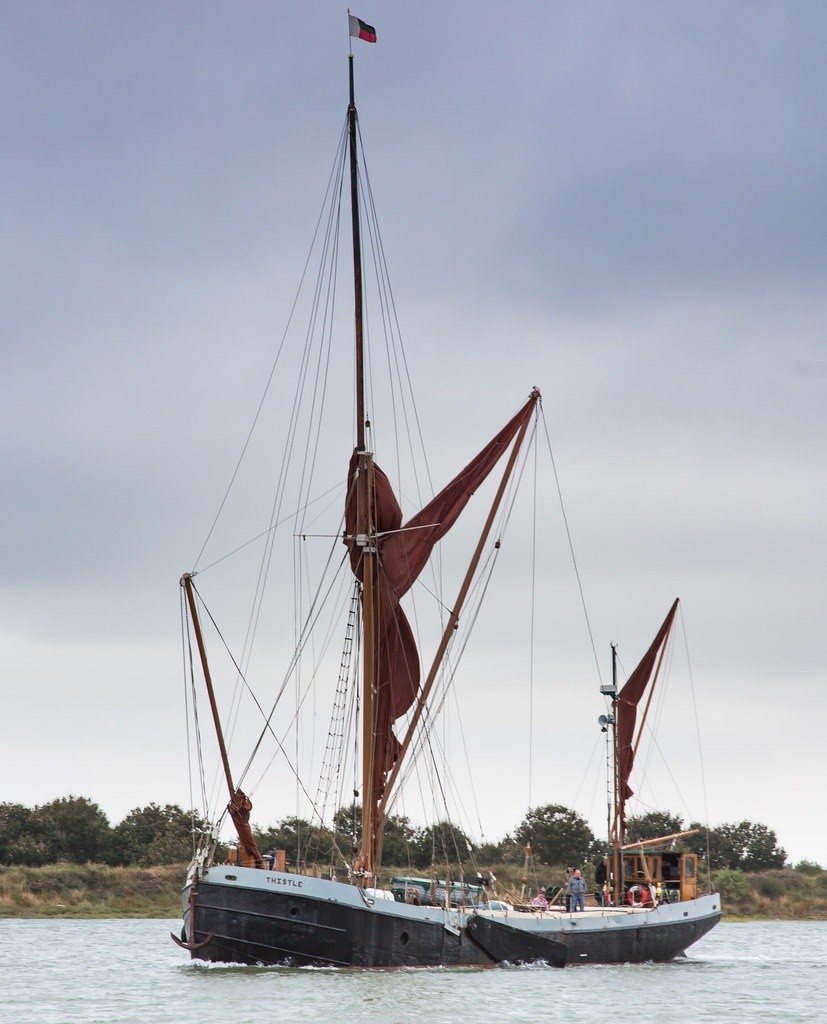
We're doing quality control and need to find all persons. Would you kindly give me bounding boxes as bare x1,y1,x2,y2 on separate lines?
532,889,548,911
594,860,607,896
565,867,586,913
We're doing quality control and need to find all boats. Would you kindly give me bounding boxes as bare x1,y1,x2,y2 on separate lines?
172,6,724,967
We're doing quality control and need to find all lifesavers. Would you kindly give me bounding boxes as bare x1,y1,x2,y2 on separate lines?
627,885,647,907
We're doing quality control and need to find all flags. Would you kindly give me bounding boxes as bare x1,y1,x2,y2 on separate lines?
349,14,378,44
